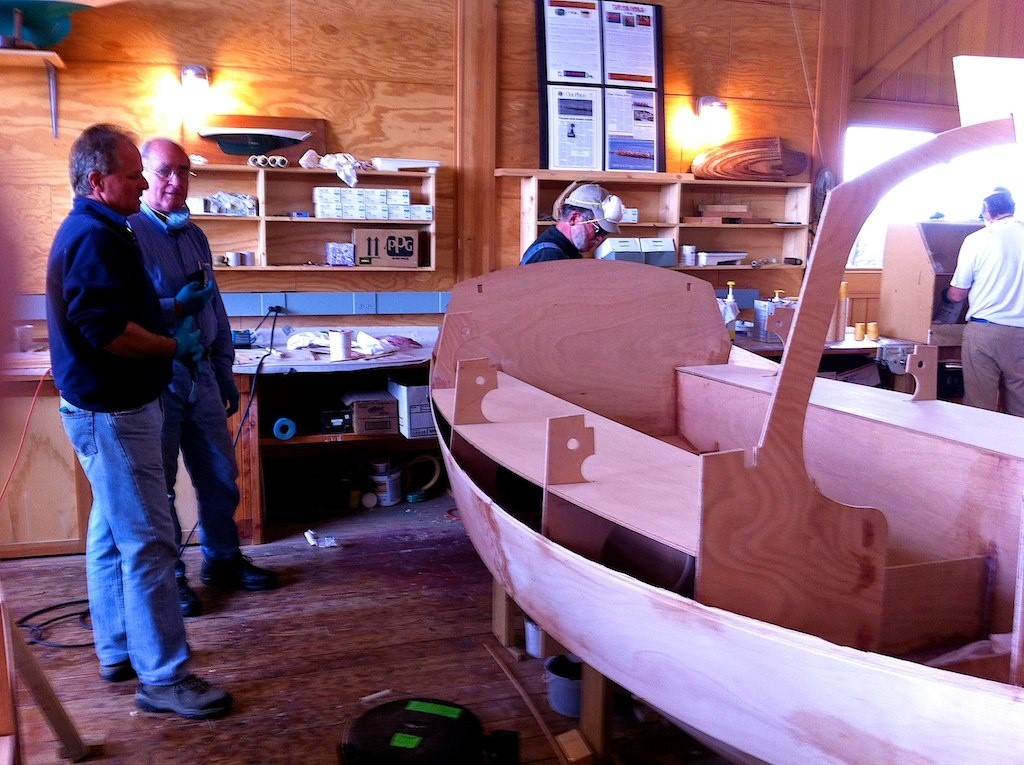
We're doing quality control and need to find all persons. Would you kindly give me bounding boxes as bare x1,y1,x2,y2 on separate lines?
47,122,235,720
944,183,1023,418
121,133,277,618
518,183,623,265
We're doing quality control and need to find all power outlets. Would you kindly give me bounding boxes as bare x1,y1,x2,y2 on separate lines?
353,292,376,314
260,292,286,316
439,292,453,313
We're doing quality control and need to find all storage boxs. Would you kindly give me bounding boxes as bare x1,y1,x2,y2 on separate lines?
351,390,399,435
387,377,438,440
352,226,418,268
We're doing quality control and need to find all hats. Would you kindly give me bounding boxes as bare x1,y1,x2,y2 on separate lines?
975,182,1009,216
565,183,622,234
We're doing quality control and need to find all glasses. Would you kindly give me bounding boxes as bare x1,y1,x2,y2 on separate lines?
577,211,608,235
143,168,197,181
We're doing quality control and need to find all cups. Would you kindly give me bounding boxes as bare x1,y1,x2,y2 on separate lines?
328,329,353,360
225,249,255,266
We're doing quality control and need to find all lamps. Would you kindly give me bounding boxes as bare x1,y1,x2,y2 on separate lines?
180,63,208,126
694,95,729,146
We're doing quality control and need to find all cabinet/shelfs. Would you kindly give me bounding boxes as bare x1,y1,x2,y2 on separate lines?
521,174,811,271
188,162,435,272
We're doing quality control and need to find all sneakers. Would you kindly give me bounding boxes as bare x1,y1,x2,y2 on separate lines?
175,576,203,617
98,642,192,683
199,557,280,591
134,675,235,719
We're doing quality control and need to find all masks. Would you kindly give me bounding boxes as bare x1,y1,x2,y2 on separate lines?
561,194,624,223
983,218,991,226
150,203,188,229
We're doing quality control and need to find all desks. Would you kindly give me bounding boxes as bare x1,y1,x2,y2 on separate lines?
2,342,434,558
724,324,926,396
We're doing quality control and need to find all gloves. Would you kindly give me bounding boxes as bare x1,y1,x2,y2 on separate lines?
172,314,202,358
941,288,952,303
189,343,203,372
219,380,239,417
174,279,215,317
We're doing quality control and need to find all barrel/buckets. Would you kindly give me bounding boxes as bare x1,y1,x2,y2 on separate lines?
368,467,403,507
